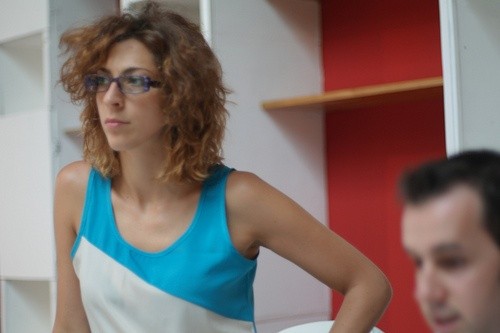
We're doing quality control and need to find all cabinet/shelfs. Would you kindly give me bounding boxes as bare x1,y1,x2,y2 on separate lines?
0,0,499,332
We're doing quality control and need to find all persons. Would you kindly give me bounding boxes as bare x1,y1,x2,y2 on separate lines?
50,4,393,331
395,149,500,333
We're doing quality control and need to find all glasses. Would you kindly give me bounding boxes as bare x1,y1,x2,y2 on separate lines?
83,74,163,94
